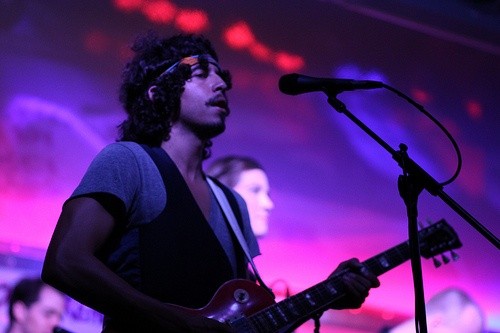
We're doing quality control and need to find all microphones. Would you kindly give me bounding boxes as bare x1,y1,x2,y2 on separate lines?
278,72,383,95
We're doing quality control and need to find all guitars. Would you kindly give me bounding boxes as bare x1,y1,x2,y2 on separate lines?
102,217,464,333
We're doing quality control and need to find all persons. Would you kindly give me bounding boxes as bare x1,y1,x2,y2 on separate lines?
41,34,380,333
208,155,274,236
8,280,64,333
381,288,482,333
